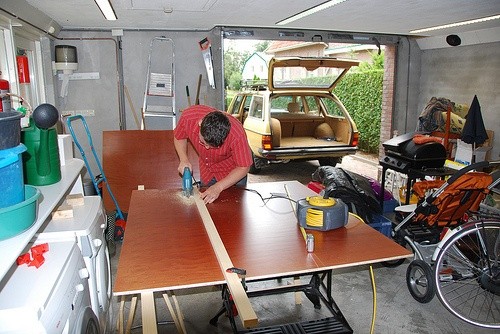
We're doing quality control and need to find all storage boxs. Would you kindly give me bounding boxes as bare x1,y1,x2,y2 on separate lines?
361,197,399,239
454,129,494,167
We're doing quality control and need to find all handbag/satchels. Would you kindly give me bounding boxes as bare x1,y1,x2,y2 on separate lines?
363,174,394,200
314,165,381,215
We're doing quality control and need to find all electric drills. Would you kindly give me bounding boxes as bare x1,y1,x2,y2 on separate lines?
184,167,194,198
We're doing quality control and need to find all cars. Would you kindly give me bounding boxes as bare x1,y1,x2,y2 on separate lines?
227,56,361,175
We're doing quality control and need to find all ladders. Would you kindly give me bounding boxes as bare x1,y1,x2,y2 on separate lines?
140,36,176,129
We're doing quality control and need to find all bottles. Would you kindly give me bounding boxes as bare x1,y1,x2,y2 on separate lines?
307,233,314,253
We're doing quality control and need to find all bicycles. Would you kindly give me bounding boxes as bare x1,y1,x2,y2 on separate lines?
432,218,499,330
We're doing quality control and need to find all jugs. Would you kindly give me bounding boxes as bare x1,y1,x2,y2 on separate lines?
20,103,62,186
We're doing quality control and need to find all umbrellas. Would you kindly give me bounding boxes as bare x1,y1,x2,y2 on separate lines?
461,95,488,143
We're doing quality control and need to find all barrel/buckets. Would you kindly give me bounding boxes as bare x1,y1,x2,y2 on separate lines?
0,143,28,209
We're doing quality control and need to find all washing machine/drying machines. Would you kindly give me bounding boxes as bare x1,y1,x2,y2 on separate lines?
0,193,114,334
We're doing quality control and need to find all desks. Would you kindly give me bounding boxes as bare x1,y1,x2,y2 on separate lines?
0,135,85,294
112,180,414,334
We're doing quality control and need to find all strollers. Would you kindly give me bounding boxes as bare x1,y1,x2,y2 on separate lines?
382,160,499,304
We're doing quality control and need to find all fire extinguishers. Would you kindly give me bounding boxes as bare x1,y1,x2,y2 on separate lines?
0,72,13,113
17,46,32,83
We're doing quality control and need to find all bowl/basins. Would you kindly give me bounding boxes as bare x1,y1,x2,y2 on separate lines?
0,184,41,242
0,110,22,150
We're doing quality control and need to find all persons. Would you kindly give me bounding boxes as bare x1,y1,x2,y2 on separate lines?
174,105,253,204
